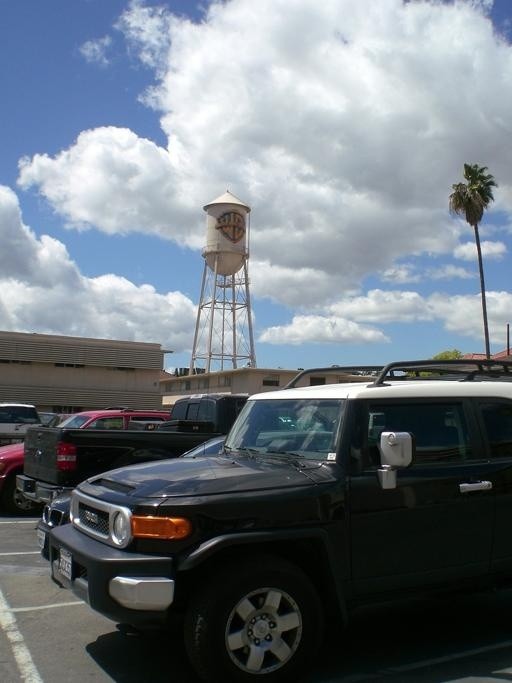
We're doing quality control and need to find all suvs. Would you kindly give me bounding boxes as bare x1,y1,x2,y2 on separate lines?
1,403,41,447
45,358,512,682
0,408,177,518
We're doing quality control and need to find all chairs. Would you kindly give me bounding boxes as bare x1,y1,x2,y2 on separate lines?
349,410,460,455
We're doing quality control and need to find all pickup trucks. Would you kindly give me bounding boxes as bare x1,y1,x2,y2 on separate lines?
14,393,261,508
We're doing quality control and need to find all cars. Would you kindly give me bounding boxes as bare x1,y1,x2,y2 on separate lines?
37,412,76,428
